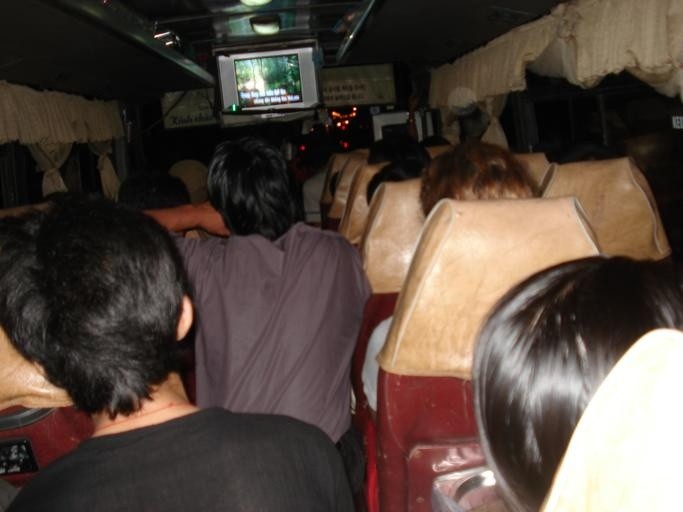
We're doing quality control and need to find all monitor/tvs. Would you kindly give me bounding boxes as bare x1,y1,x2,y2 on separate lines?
216,46,320,113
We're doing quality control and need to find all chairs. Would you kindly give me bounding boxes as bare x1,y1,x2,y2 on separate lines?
0,142,682,511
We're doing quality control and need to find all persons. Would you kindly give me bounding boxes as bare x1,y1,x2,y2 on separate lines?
1,136,373,511
470,255,683,512
361,135,537,413
